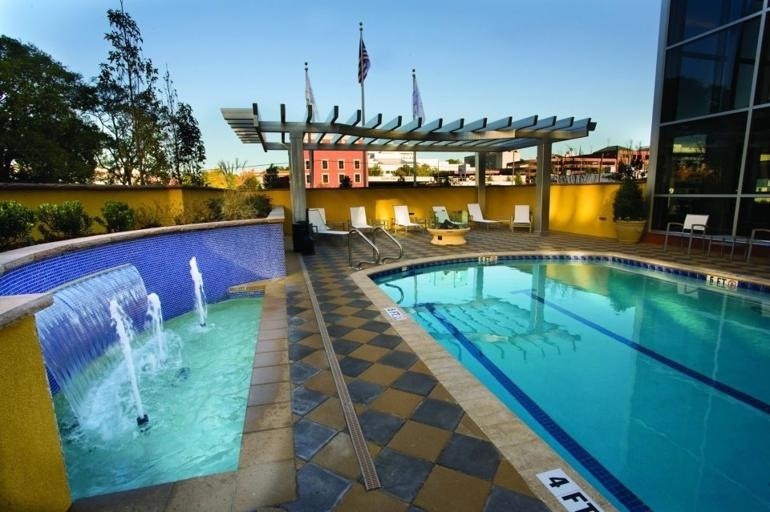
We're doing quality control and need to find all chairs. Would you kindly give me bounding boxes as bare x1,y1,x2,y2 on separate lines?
664,214,710,259
307,203,537,246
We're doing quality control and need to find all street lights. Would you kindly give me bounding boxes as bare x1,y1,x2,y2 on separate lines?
512,150,517,183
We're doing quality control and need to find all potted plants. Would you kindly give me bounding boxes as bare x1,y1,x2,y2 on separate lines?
609,175,648,244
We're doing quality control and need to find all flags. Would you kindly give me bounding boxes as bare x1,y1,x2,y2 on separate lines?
357,32,370,84
412,78,426,126
304,73,319,123
